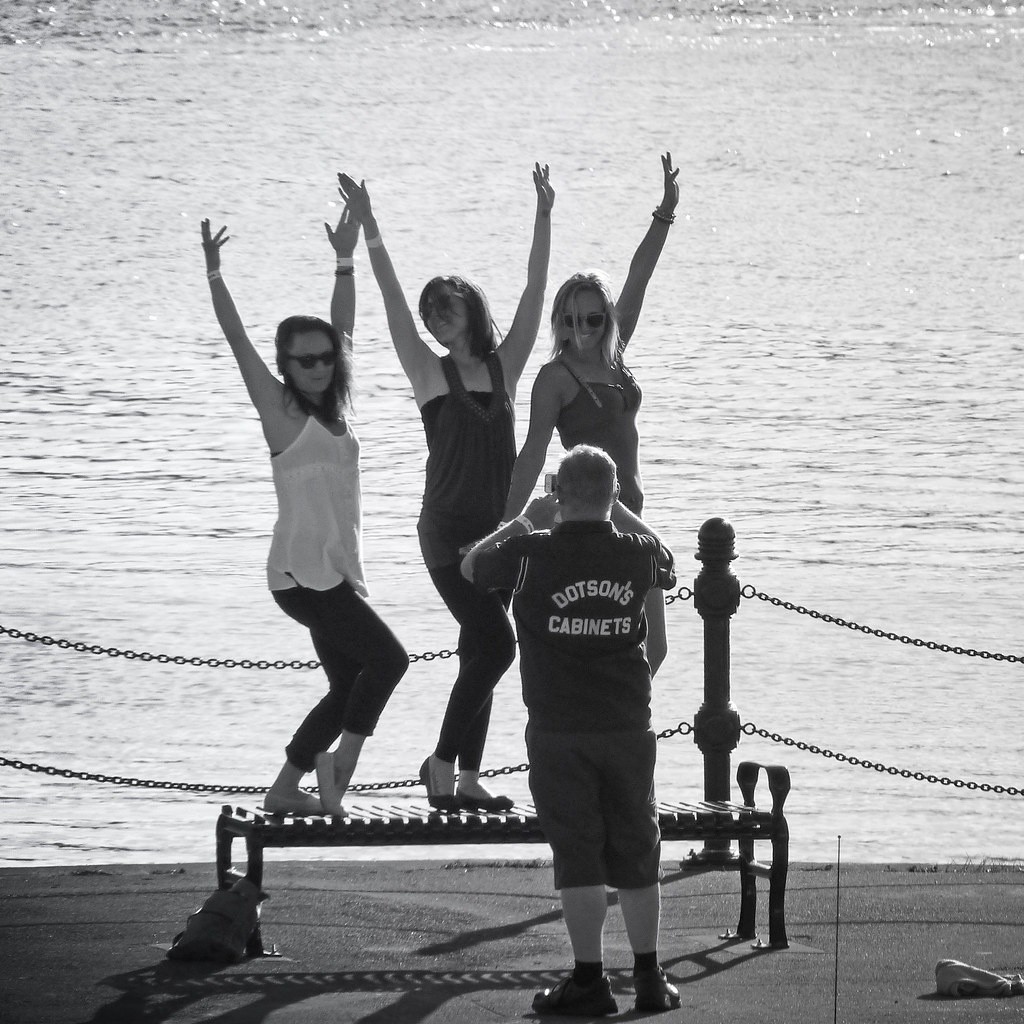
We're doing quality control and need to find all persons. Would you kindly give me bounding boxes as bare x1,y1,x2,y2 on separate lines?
459,445,684,1016
201,204,408,815
453,152,678,680
338,159,556,813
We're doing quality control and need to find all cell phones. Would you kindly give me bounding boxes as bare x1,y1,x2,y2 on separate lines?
544,474,556,493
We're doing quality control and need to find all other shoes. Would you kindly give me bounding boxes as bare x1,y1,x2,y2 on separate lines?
454,788,514,811
419,756,455,809
264,791,327,813
317,751,348,816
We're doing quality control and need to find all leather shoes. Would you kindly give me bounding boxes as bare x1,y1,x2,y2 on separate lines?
533,976,619,1015
635,967,683,1012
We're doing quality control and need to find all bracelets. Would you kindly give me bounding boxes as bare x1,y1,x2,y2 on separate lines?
651,206,676,224
207,270,222,281
333,257,355,277
515,515,534,535
366,234,384,248
497,521,507,529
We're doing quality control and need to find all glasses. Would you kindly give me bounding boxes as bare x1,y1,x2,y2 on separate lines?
560,312,607,329
419,291,467,320
287,351,339,370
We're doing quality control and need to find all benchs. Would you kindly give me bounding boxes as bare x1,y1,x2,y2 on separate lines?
215,762,790,958
